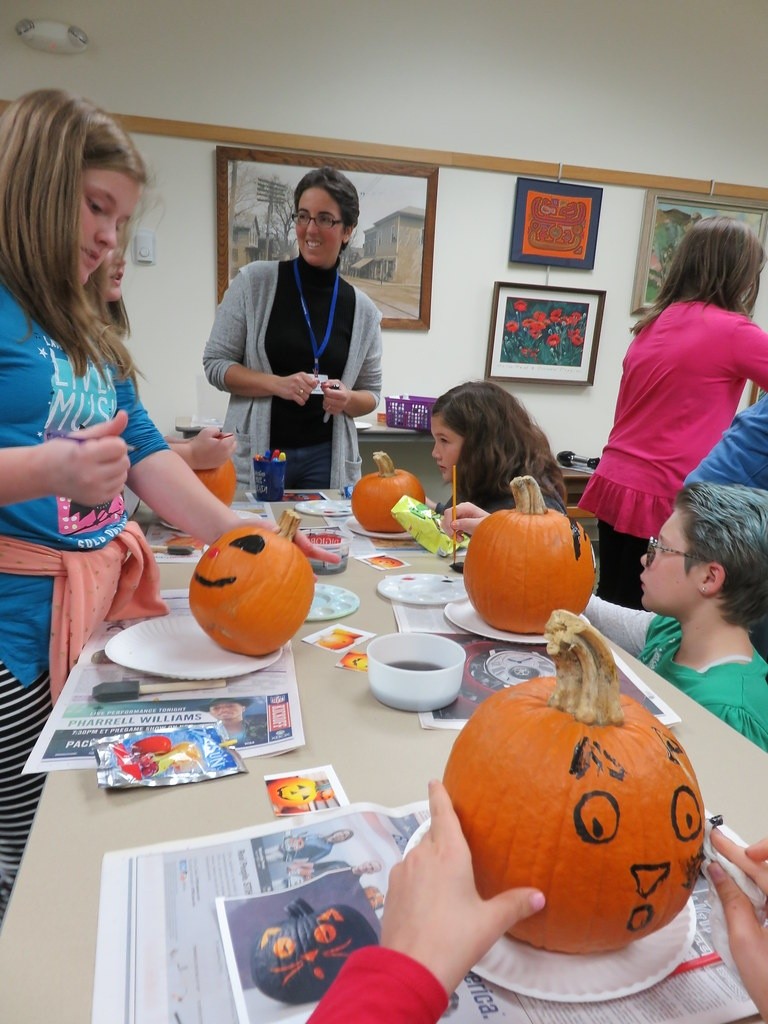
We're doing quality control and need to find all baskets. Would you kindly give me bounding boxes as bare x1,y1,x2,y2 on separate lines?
384,395,438,433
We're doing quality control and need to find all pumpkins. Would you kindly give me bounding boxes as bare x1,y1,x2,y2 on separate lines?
188,508,315,656
440,608,707,956
463,476,597,636
351,451,426,533
193,458,235,507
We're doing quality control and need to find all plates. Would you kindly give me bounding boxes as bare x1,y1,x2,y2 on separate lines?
305,583,360,623
226,509,264,525
293,497,352,518
444,599,556,646
377,571,473,606
346,510,416,541
469,894,695,1004
99,611,288,684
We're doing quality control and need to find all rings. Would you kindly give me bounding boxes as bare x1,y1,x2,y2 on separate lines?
299,390,303,394
326,405,331,410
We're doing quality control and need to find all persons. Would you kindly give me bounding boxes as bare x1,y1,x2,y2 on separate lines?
0,88,342,922
304,781,768,1024
203,168,384,490
265,827,382,891
578,221,768,612
199,698,269,751
100,244,134,340
683,393,768,491
423,380,567,545
440,483,768,752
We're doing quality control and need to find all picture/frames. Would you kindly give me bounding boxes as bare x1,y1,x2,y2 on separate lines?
633,194,768,321
509,177,603,268
206,147,440,329
485,282,608,387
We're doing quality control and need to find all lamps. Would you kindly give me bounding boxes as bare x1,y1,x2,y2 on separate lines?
14,19,88,55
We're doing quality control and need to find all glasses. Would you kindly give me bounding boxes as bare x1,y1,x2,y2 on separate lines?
646,536,710,567
292,213,343,229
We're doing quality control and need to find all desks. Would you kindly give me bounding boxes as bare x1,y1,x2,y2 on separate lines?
163,419,435,444
0,486,768,1024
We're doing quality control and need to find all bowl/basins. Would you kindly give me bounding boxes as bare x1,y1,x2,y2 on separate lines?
355,629,469,714
